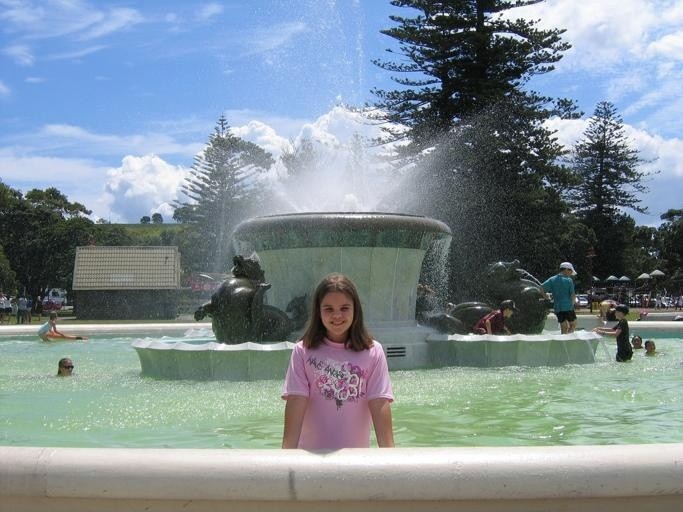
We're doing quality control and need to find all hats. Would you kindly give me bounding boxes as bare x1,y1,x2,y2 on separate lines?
610,304,629,314
500,300,520,312
560,262,578,275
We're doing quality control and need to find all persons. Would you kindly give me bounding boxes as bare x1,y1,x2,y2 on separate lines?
588,304,634,363
472,299,521,335
588,284,683,313
538,261,579,334
631,334,644,350
36,311,65,344
279,270,395,449
57,358,76,377
645,340,658,357
0,292,44,324
